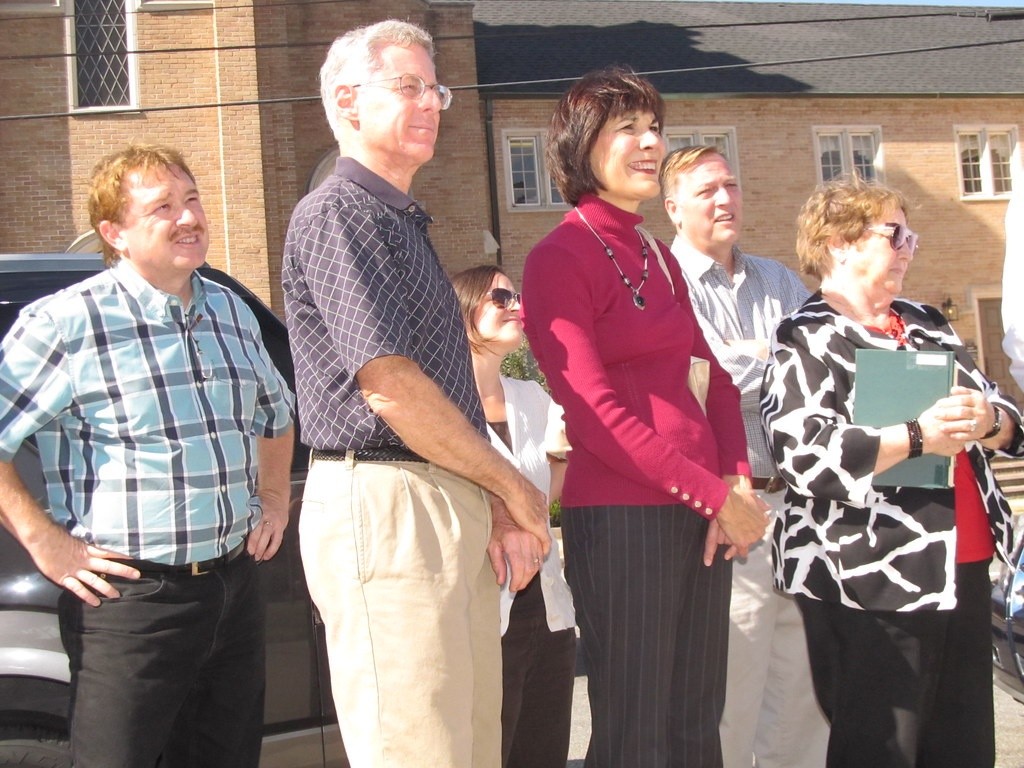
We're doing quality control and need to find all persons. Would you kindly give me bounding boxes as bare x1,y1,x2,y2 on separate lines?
279,18,553,768
756,170,1024,768
659,142,830,768
0,142,297,768
521,64,773,768
443,266,571,768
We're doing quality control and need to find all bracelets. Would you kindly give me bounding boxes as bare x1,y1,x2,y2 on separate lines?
903,415,925,460
978,400,1004,439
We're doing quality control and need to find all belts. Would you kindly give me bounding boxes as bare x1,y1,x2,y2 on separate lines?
106,539,246,576
311,445,430,463
752,476,788,494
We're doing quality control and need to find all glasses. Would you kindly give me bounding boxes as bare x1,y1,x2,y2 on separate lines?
351,74,453,110
862,223,919,251
484,288,520,309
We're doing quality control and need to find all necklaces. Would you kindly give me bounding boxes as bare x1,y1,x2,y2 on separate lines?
573,205,651,314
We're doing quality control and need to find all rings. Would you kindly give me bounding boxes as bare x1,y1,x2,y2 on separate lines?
533,557,541,564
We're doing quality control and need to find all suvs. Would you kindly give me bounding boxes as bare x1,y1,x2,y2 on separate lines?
0,251,345,768
